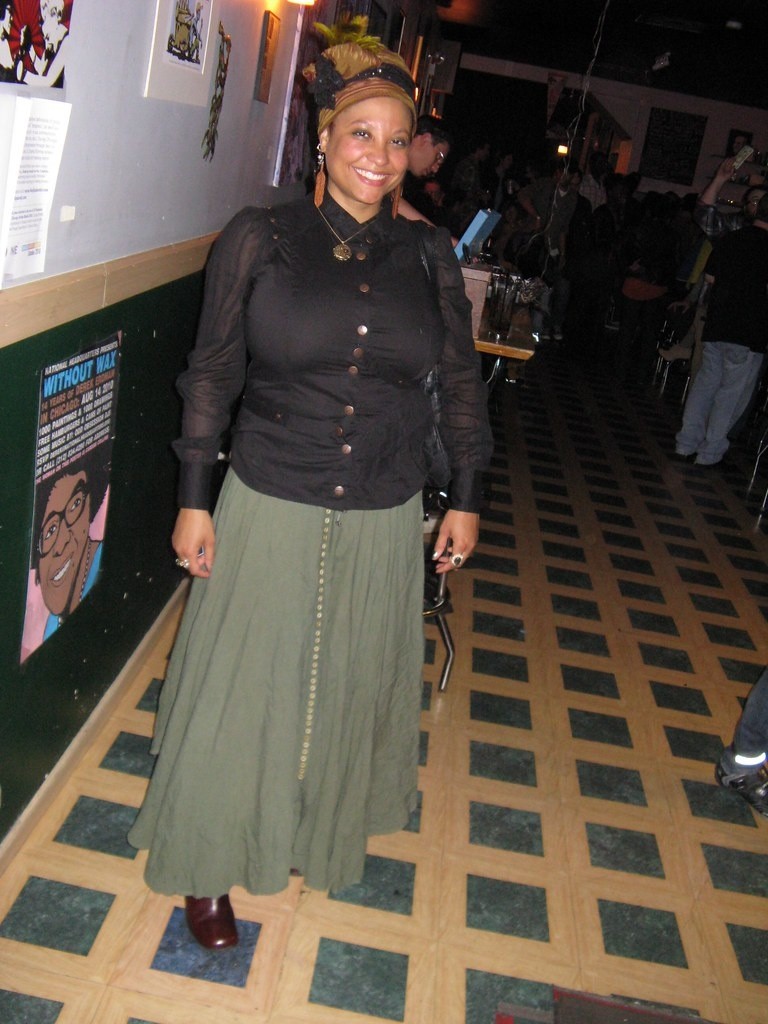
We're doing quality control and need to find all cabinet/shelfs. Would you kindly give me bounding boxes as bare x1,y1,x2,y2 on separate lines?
705,152,767,207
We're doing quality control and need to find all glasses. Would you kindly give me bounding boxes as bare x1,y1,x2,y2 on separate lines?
743,198,759,206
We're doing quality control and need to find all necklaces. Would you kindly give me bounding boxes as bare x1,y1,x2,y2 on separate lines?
316,202,380,261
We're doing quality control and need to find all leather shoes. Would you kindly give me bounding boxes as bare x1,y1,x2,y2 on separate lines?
184,896,237,950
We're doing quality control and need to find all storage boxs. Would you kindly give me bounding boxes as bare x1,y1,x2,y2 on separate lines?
454,207,502,339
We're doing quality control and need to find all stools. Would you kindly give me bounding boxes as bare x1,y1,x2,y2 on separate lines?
424,513,455,692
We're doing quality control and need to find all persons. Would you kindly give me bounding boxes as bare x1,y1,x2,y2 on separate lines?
128,35,496,953
666,190,767,472
404,139,725,382
400,112,463,263
689,158,768,388
714,668,767,817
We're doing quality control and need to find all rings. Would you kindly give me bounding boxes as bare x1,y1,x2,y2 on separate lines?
451,553,463,567
176,559,189,568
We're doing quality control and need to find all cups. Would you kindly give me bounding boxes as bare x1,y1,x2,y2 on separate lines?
507,179,517,195
489,272,521,336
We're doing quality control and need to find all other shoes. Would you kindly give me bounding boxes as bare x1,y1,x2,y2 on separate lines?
716,758,768,817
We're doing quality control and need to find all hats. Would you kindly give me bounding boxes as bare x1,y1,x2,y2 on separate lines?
302,42,417,138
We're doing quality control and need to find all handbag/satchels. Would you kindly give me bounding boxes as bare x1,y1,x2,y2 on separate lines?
424,367,453,486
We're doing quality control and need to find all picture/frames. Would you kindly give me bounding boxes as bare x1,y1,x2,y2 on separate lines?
367,0,387,46
725,128,754,157
252,10,281,105
390,7,407,56
143,0,222,106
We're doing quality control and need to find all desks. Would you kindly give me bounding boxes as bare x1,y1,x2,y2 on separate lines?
474,305,534,417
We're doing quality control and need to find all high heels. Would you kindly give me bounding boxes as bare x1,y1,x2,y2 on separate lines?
657,343,691,368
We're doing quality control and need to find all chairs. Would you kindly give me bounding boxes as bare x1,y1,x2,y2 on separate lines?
652,305,768,511
